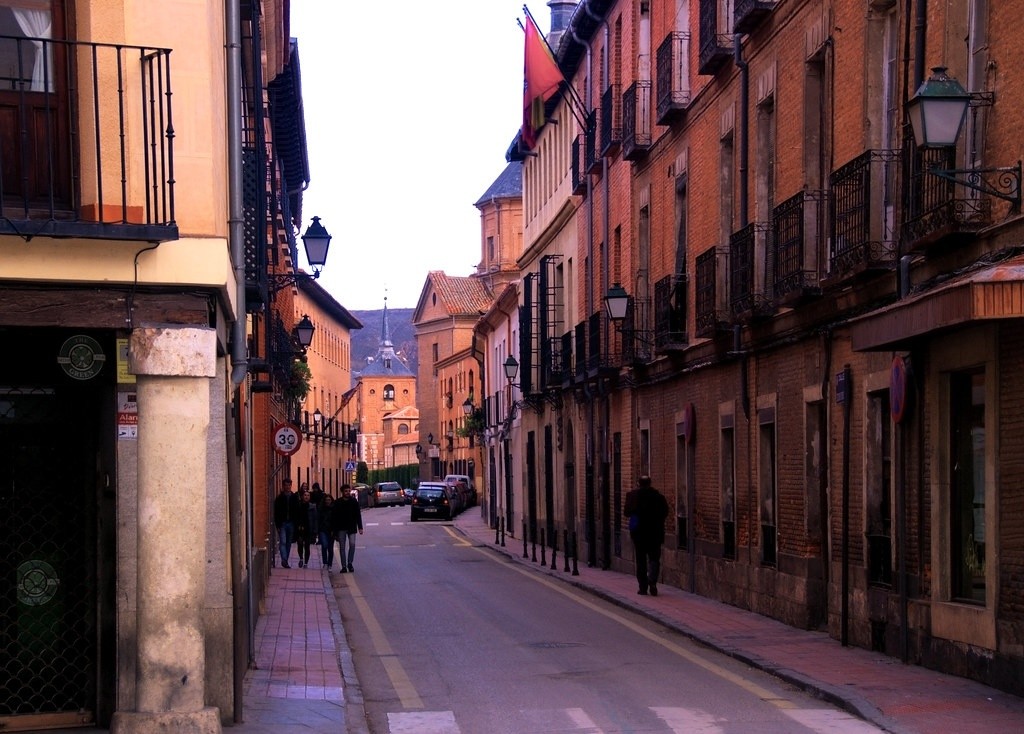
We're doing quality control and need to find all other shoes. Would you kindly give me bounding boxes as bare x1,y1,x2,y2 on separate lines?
281,560,291,568
648,580,657,596
347,563,354,572
637,590,647,595
328,566,332,573
316,541,320,545
304,565,308,569
340,566,348,573
323,564,329,573
299,559,303,568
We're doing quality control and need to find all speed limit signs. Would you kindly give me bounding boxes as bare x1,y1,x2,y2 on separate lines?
271,421,303,458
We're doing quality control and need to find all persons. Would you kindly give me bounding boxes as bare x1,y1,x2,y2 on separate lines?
309,481,326,546
330,484,364,574
316,493,337,571
272,477,299,568
622,475,669,597
292,490,318,569
294,482,311,509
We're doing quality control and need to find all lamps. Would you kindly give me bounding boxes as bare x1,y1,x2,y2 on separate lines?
416,443,426,454
278,314,316,351
462,398,481,419
428,433,440,445
348,419,360,434
905,67,1023,208
268,215,333,294
503,354,536,392
448,426,457,437
302,408,321,427
604,281,667,346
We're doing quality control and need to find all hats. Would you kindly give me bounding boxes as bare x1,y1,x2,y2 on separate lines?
312,483,319,489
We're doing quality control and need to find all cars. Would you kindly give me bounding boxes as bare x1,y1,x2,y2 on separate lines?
368,481,405,508
403,473,477,523
